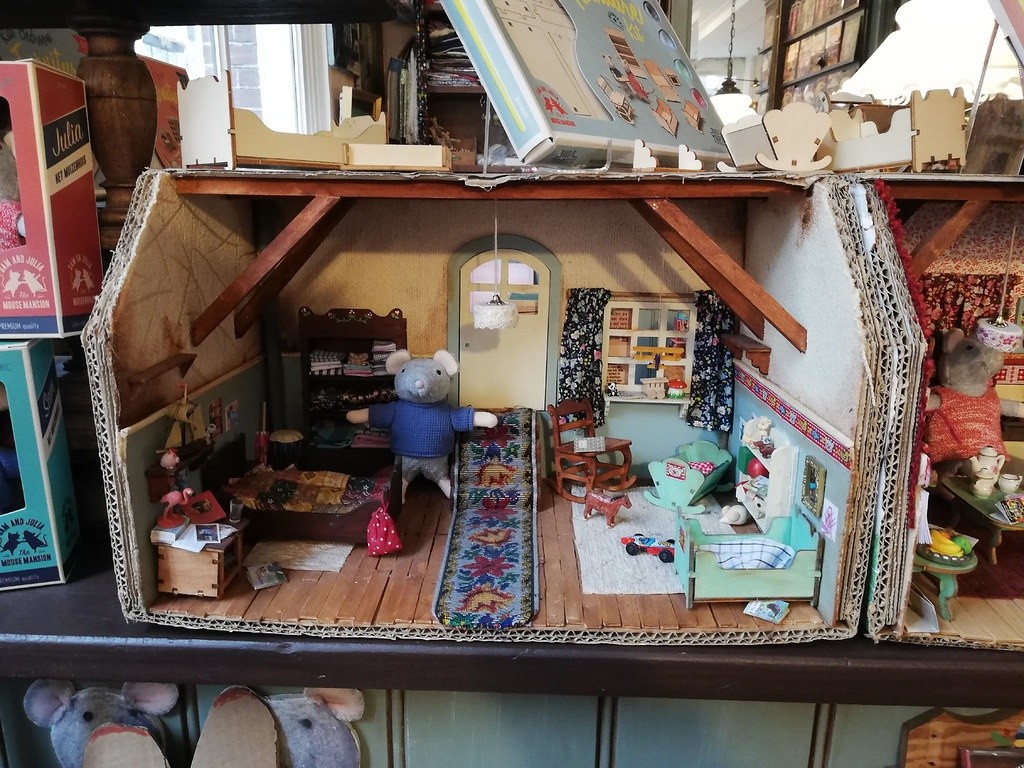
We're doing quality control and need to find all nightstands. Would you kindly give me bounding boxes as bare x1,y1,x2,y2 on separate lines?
152,518,251,599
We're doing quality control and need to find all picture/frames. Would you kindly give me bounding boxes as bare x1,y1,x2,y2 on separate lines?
801,455,827,518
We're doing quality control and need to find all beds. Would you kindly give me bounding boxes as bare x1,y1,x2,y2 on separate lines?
674,505,826,610
644,440,734,515
200,433,402,544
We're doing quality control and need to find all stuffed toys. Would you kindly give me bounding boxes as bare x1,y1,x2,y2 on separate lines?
161,451,181,469
346,349,499,504
927,329,1024,481
23,679,364,768
736,480,767,519
606,382,618,397
742,417,770,448
719,506,749,525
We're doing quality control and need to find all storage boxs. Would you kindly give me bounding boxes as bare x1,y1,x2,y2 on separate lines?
0,342,84,591
439,0,738,174
1,58,106,339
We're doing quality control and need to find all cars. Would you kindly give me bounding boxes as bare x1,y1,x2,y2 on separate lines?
620,532,675,564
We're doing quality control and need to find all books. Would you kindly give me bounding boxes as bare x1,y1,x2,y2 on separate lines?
151,515,190,540
246,561,288,590
743,600,790,625
574,437,605,453
990,498,1024,525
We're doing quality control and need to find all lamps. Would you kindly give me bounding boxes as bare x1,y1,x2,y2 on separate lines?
475,199,521,330
973,201,1023,354
706,1,752,122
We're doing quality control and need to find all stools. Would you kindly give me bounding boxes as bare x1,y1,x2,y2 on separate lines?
912,553,978,621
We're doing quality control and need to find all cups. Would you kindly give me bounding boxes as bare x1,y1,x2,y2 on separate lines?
999,474,1023,493
971,469,994,498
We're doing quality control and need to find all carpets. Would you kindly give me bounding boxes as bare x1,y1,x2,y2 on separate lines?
242,539,355,573
432,407,540,630
570,484,738,595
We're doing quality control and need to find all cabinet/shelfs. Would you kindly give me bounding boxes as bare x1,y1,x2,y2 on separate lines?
736,416,799,535
417,3,526,174
297,306,407,468
756,0,902,116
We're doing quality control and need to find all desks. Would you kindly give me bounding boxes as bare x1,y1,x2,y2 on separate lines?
937,476,1024,564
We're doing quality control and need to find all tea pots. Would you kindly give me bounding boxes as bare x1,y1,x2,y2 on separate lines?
968,446,1005,485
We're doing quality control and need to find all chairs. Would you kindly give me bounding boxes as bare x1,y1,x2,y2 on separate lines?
546,398,637,502
